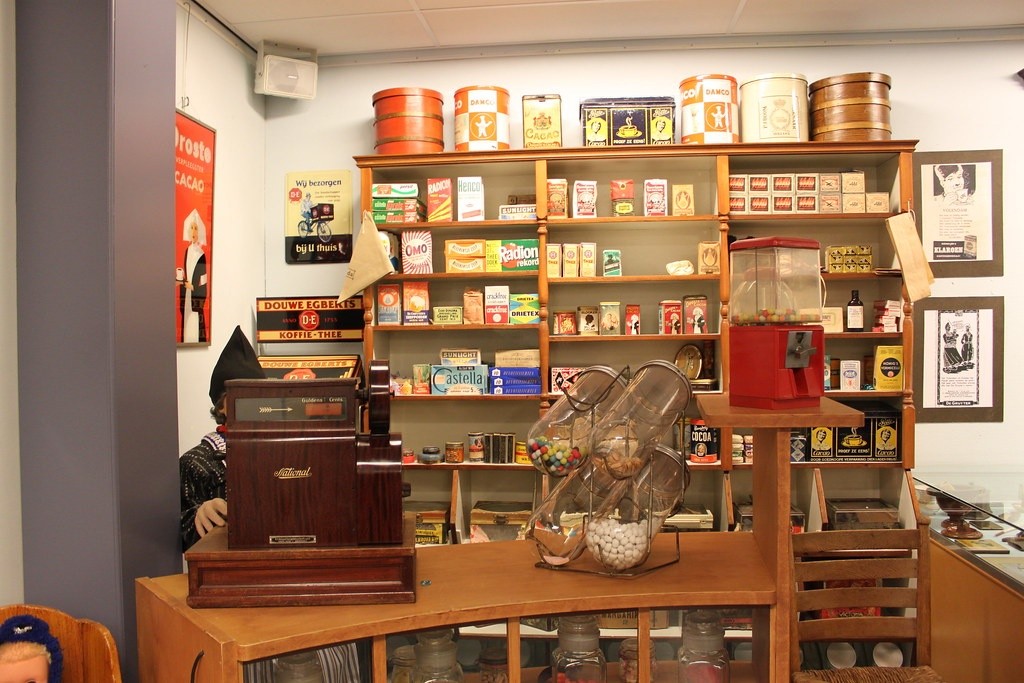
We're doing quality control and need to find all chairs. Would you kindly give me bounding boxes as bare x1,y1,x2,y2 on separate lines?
785,516,945,682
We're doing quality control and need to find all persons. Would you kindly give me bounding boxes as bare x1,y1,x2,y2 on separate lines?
179,325,360,683
181,208,207,341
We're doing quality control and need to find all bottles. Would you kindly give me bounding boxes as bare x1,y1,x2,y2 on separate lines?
273,651,325,683
548,613,732,683
389,631,508,683
846,289,865,332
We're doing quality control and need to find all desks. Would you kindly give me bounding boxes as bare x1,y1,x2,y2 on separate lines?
138,528,775,683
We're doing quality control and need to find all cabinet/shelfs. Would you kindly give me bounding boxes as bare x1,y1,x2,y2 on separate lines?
350,142,927,640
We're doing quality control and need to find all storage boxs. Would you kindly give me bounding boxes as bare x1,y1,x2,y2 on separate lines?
579,96,676,146
374,171,917,629
523,95,563,147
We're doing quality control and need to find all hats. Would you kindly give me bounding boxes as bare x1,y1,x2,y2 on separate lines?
209,326,267,407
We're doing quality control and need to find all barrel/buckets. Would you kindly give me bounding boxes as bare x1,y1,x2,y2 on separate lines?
371,87,445,154
452,85,510,152
806,72,894,142
677,73,742,145
738,72,812,143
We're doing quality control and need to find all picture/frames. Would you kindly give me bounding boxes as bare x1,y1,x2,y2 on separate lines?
912,292,1005,423
172,108,216,346
911,146,1005,279
